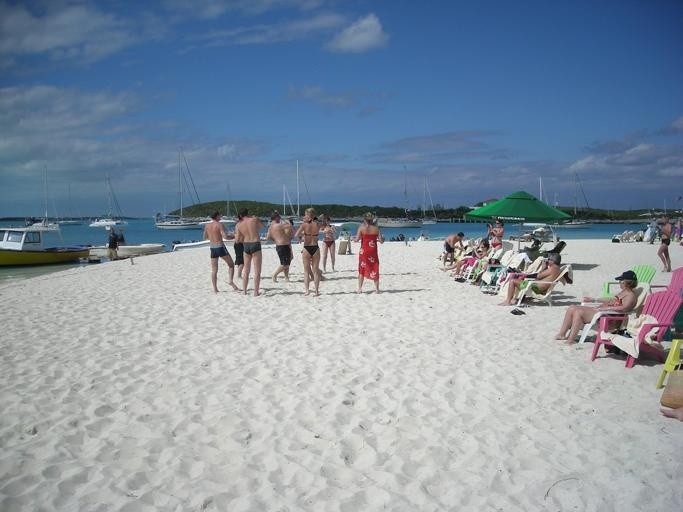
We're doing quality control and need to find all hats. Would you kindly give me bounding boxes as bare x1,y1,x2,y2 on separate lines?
615,271,637,280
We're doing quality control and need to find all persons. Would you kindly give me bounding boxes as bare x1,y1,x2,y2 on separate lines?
204,210,238,293
649,213,683,241
264,220,294,241
352,212,385,295
322,218,337,274
439,220,561,306
655,215,672,272
294,207,323,297
265,212,293,283
553,270,638,346
233,218,244,278
235,207,263,297
107,229,119,262
659,406,683,423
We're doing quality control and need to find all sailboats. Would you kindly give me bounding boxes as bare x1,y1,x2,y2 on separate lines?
516,170,683,229
153,148,244,231
88,175,128,228
281,160,311,227
29,164,62,231
374,166,426,229
422,174,437,225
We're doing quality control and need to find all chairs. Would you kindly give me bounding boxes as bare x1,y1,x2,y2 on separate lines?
602,264,656,297
613,230,644,242
591,289,683,368
668,266,683,295
657,339,683,389
440,238,570,308
578,283,650,345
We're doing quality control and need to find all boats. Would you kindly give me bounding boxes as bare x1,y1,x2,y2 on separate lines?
87,236,267,258
0,221,89,267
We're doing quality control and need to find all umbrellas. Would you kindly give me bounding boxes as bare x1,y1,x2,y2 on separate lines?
464,190,573,249
